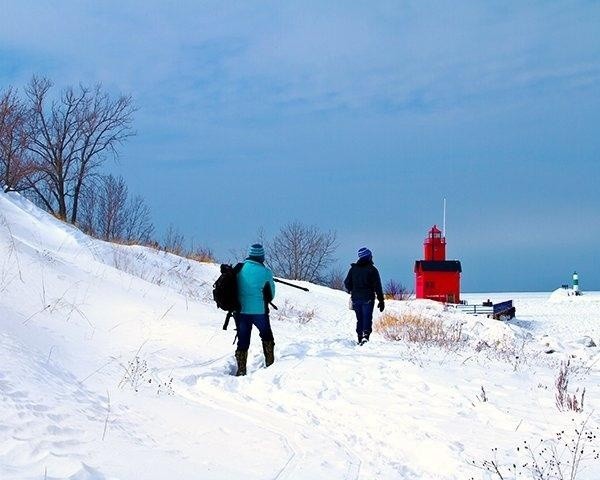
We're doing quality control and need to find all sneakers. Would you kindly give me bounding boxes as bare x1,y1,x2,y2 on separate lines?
357,330,371,346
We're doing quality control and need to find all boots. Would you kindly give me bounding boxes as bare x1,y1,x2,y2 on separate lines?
234,350,248,376
262,338,275,368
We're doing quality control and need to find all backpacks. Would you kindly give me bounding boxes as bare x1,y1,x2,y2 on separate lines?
212,262,245,315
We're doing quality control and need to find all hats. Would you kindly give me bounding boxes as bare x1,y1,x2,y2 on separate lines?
356,247,373,262
246,243,267,262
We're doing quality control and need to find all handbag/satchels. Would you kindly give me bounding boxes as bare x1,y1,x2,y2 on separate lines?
348,295,356,310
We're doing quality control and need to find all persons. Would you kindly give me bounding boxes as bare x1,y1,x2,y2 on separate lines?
343,247,385,346
233,244,276,376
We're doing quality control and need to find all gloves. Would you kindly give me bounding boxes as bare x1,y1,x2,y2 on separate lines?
377,300,385,312
